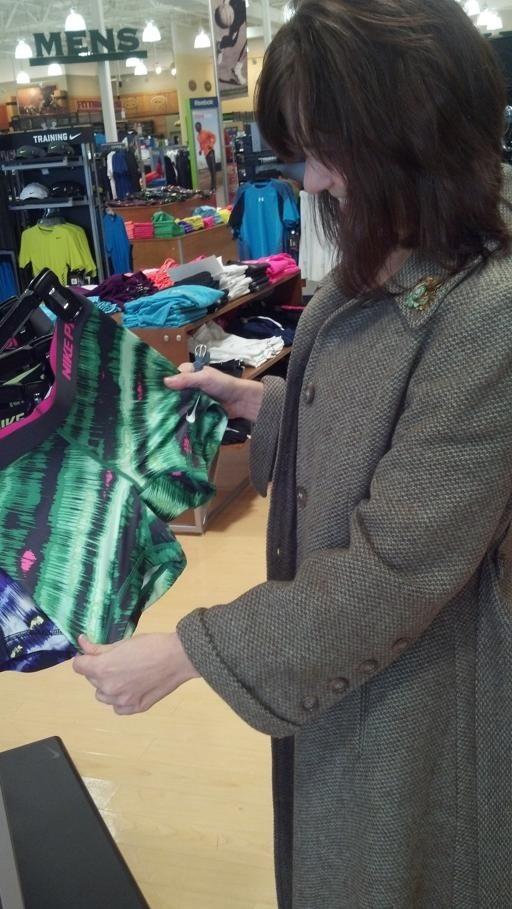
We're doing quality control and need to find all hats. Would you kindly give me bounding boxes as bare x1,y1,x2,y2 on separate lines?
18,181,49,201
48,179,83,197
13,144,46,159
45,139,75,156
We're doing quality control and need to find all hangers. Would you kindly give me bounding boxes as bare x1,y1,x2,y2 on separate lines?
1,267,55,362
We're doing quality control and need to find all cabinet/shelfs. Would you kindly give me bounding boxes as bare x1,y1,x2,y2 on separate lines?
0,125,109,286
101,254,307,541
111,192,239,274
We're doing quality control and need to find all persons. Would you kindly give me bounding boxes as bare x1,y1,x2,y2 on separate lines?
195,122,217,194
72,0,512,908
215,0,247,85
